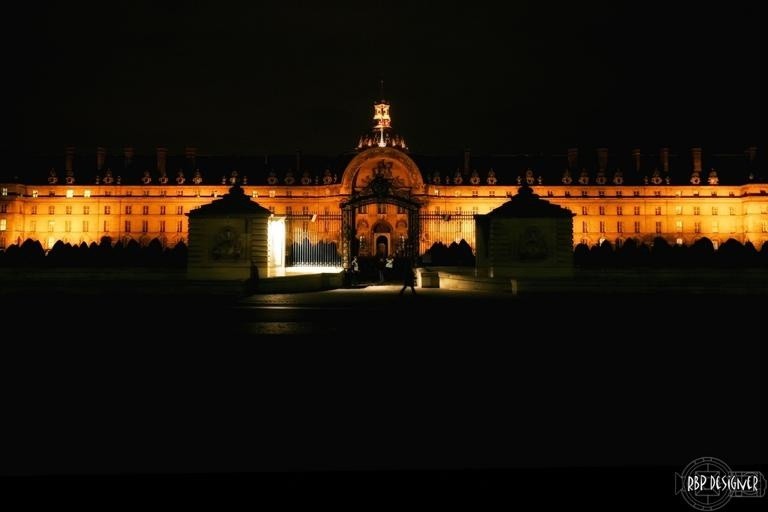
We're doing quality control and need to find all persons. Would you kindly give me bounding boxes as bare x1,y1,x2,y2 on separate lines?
351,256,359,287
250,260,258,280
400,264,415,294
385,254,394,269
